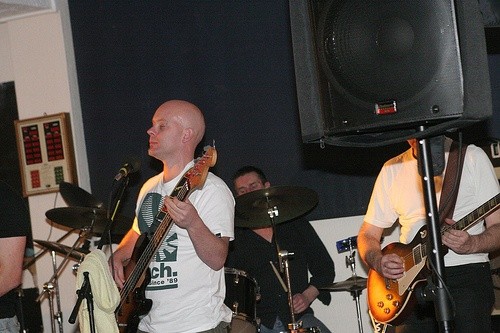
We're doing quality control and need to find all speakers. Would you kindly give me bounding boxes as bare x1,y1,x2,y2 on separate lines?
288,0,500,148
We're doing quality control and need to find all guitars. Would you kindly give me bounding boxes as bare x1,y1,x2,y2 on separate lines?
366,193,500,326
114,138,219,333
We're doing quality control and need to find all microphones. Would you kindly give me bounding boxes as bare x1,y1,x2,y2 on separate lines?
114,163,133,182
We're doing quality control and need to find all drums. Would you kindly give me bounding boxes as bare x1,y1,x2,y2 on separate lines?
222,267,262,333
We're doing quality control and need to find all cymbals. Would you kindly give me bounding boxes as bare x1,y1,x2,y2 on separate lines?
33,238,86,263
59,181,104,210
234,184,320,229
320,275,368,291
45,205,134,235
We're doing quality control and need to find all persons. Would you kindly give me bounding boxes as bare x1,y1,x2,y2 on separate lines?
357,124,500,333
223,159,336,333
0,146,29,333
109,99,237,333
